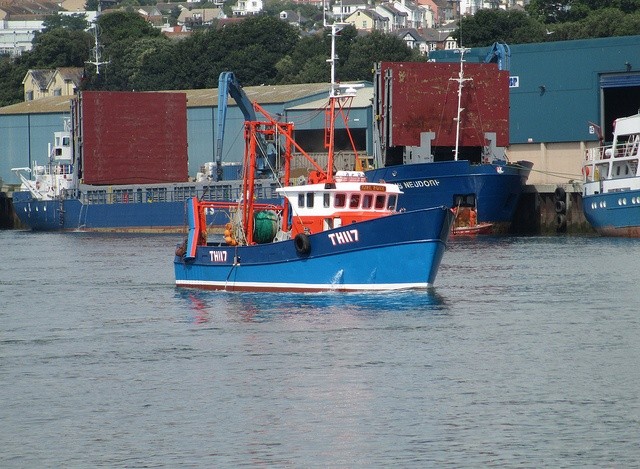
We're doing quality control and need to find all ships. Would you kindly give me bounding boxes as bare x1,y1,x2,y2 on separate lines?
582,111,640,236
365,45,535,234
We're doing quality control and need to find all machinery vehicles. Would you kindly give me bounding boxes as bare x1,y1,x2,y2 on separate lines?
212,71,289,182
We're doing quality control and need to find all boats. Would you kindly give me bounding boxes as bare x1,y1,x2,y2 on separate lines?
173,191,455,293
276,0,405,238
